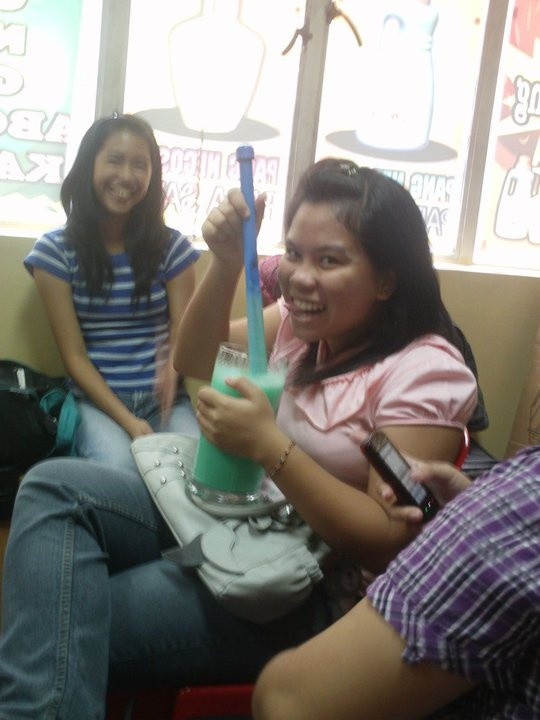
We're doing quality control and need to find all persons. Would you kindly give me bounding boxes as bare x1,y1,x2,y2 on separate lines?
0,160,479,720
251,428,540,720
20,112,203,483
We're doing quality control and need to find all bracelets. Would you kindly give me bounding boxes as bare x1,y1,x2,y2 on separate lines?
266,439,297,479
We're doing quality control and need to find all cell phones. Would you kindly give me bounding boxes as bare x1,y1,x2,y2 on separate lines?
360,430,439,521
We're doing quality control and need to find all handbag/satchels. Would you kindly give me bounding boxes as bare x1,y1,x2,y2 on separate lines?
126,432,327,624
0,358,66,498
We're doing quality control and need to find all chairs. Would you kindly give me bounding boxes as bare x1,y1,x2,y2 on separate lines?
112,424,473,720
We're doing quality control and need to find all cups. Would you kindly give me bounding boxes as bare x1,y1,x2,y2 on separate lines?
184,346,289,507
